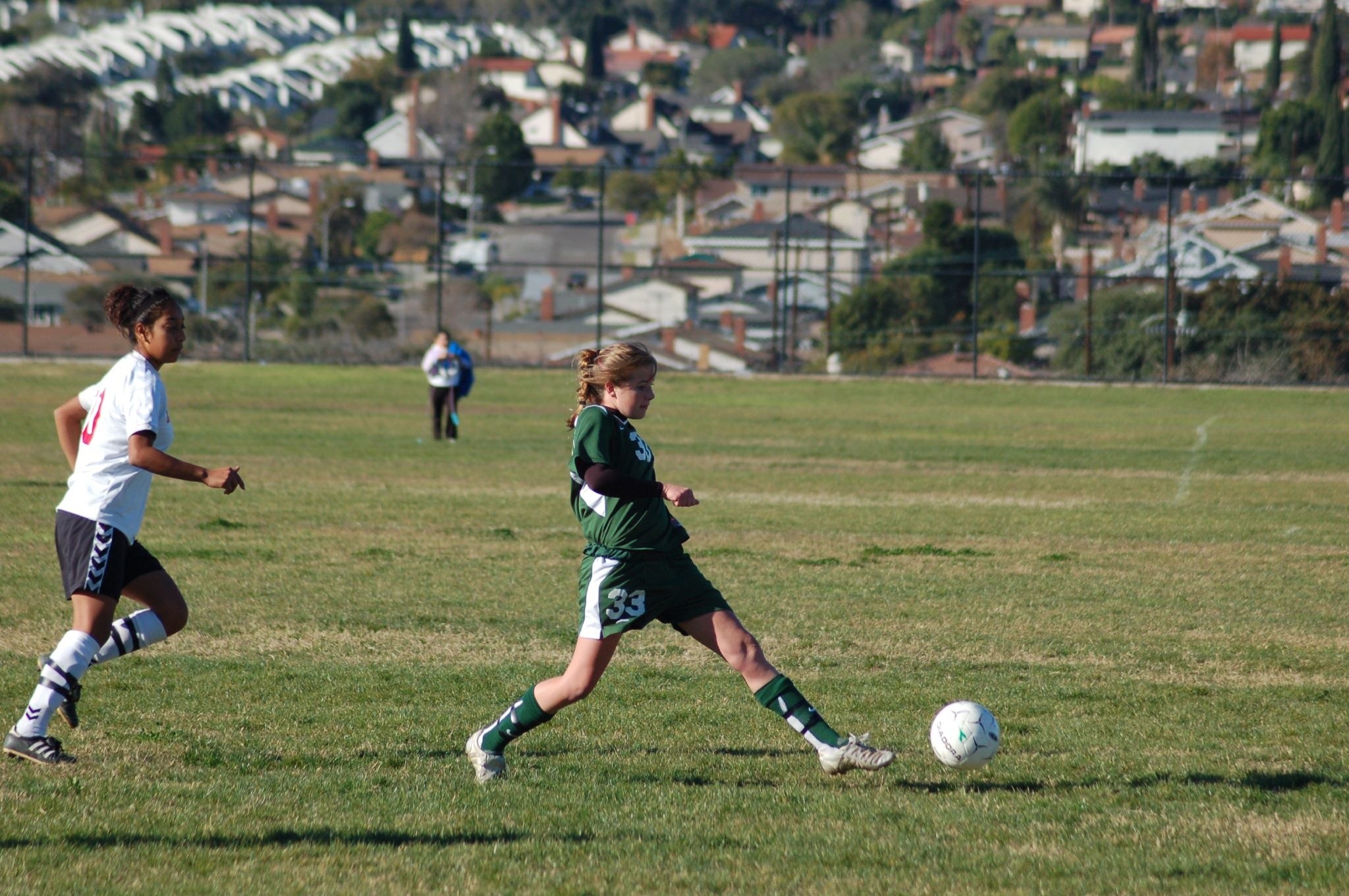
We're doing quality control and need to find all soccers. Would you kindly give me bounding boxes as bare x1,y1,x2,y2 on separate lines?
931,702,999,770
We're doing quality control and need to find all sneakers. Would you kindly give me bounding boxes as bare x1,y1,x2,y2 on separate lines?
3,725,77,766
36,653,83,729
819,732,895,776
465,729,507,786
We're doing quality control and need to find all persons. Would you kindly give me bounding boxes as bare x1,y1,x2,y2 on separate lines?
2,283,245,766
464,340,895,794
419,330,463,445
